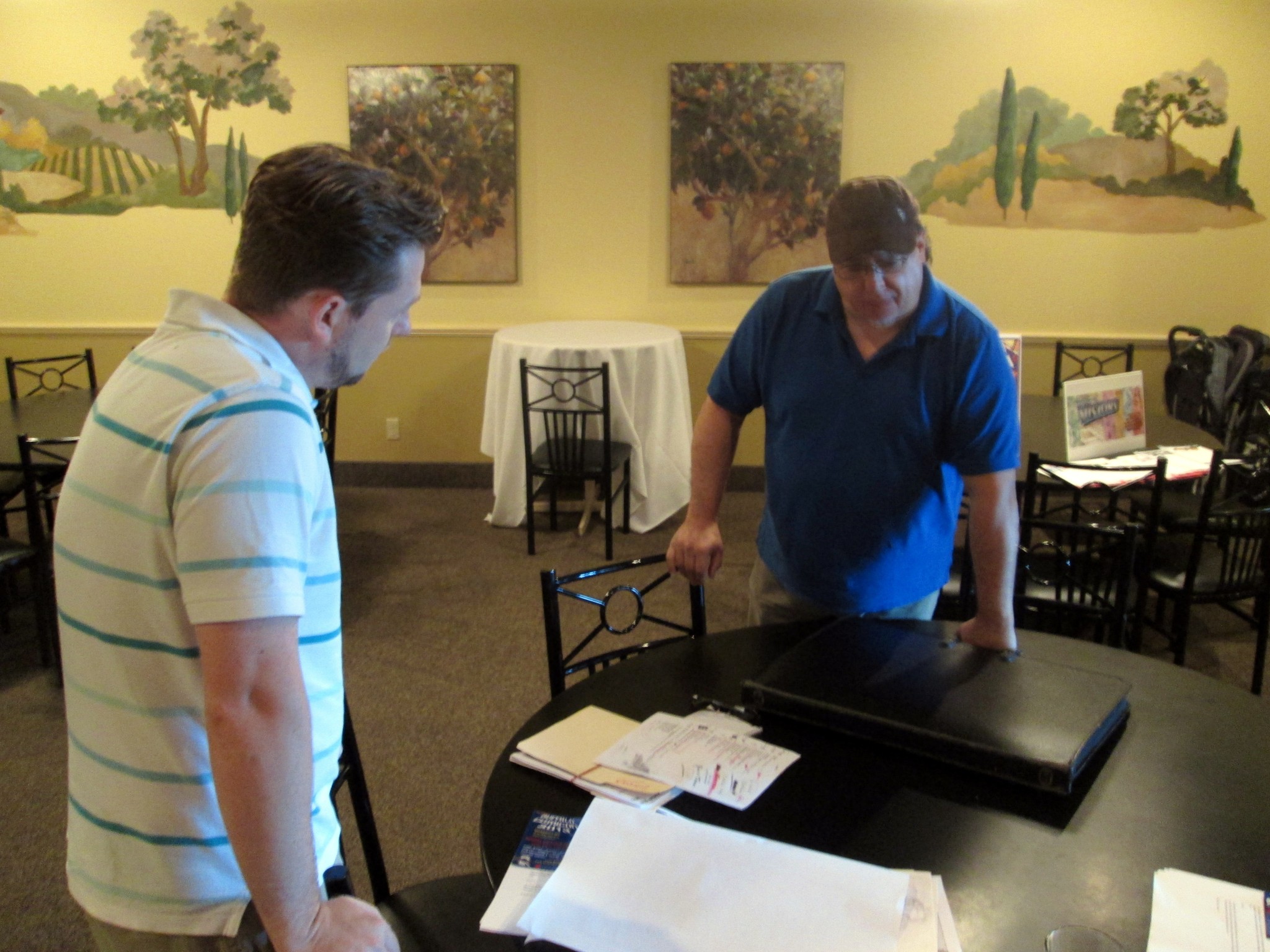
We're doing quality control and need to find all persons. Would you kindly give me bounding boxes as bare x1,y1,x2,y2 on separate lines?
667,177,1021,653
52,143,450,952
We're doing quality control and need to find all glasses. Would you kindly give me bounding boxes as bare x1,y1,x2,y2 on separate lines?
832,255,908,280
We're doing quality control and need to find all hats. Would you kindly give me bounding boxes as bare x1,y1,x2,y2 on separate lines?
827,177,920,260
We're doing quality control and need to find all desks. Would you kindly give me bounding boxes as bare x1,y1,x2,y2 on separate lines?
0,387,100,531
481,616,1270,952
962,394,1228,650
480,321,694,534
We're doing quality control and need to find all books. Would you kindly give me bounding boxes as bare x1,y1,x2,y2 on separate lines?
478,704,962,952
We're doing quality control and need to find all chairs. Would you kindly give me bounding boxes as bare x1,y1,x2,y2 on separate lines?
0,348,96,668
936,327,1270,697
540,554,708,701
330,688,509,952
520,358,632,562
